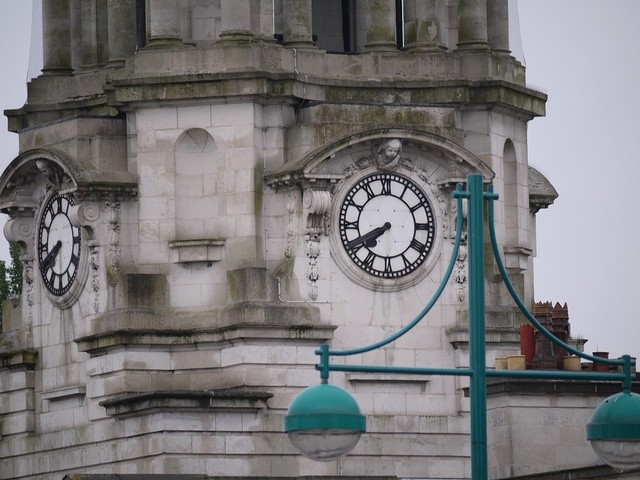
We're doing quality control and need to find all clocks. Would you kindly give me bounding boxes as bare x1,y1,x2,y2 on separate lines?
32,183,92,309
325,155,449,294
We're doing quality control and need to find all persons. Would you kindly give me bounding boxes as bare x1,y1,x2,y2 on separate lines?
377,138,403,173
34,158,62,192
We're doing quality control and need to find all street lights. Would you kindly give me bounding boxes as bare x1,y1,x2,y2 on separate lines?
283,171,640,480
